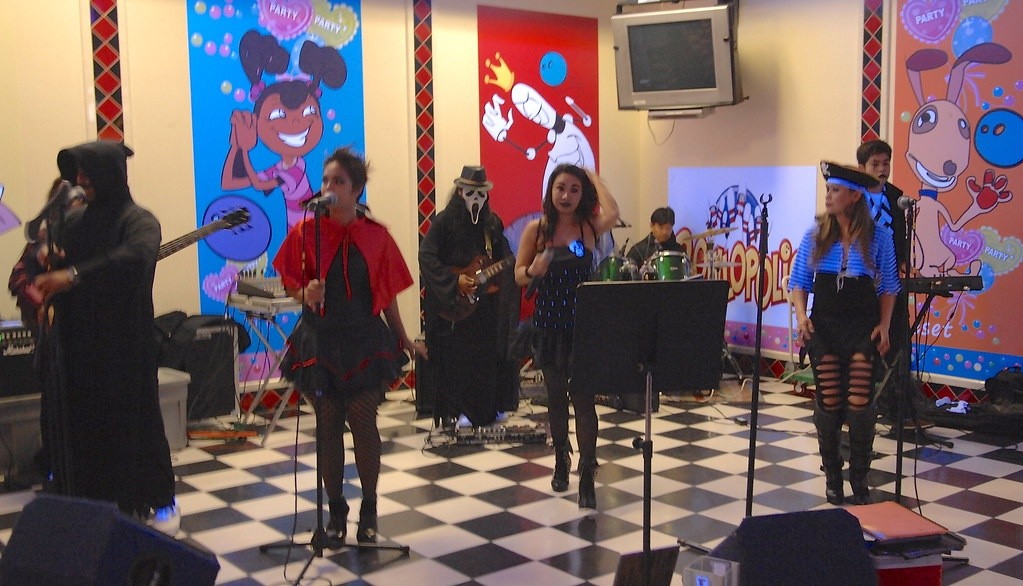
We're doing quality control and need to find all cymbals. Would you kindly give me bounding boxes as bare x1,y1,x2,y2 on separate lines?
683,226,739,241
695,262,742,268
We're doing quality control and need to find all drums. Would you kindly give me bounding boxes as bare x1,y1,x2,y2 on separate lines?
648,251,690,280
590,254,637,282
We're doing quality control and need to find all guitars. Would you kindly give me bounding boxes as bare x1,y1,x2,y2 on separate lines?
420,251,517,323
16,205,253,340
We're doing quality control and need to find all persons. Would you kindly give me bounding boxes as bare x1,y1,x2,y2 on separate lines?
25,142,175,537
624,209,690,282
514,164,620,510
8,175,86,500
417,166,520,441
272,150,428,553
857,140,919,420
787,160,901,506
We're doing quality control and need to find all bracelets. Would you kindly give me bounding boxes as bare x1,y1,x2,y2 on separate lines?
404,339,410,349
66,263,81,283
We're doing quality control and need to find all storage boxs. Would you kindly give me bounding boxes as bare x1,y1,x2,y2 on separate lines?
683,556,740,586
157,367,190,448
871,551,943,586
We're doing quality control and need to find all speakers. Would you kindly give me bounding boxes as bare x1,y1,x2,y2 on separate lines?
0,320,44,400
0,496,221,586
709,508,878,586
159,323,241,428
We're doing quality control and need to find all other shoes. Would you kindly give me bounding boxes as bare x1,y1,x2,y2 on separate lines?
151,501,181,538
496,410,508,422
456,413,473,431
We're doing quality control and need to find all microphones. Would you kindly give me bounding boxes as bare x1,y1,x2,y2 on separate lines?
55,186,87,211
897,195,916,209
619,238,629,257
525,249,553,299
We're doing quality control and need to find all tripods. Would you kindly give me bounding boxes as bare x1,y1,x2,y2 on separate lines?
259,202,410,586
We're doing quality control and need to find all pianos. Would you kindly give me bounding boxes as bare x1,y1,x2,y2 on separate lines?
812,274,985,448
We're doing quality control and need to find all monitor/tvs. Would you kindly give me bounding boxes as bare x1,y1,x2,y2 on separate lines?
611,4,733,110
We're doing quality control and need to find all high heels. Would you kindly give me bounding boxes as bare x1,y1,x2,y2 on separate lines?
357,499,379,543
326,496,349,548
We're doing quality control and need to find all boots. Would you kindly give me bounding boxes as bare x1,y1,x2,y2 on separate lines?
578,455,600,508
847,408,877,504
551,436,573,491
813,398,844,506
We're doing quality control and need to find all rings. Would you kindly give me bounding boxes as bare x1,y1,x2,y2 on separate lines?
797,329,801,334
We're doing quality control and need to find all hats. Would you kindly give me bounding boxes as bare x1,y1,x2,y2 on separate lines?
819,160,879,195
454,165,493,192
